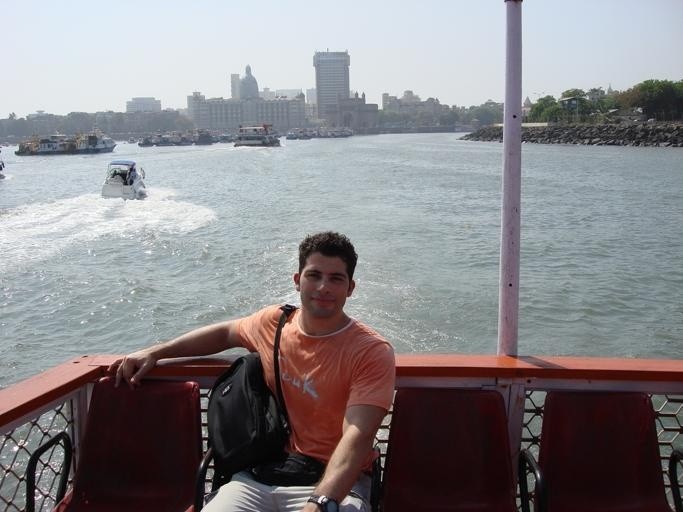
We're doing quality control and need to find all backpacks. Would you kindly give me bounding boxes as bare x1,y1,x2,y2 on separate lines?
241,452,326,487
206,303,297,475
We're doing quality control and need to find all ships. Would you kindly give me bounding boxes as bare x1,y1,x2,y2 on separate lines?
137,127,222,146
286,126,354,140
14,127,117,156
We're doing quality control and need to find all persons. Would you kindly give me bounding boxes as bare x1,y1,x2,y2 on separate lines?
106,231,395,512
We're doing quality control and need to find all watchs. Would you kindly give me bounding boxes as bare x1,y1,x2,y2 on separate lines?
307,493,339,512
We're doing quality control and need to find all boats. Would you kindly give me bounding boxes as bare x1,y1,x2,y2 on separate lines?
233,121,282,147
128,137,137,143
101,160,145,199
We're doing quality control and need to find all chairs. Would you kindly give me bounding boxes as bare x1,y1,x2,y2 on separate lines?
371,386,546,512
536,389,683,511
27,375,219,511
